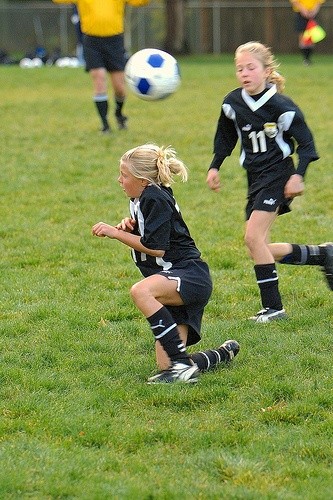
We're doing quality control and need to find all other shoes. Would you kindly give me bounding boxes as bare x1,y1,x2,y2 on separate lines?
119,117,128,128
100,125,109,132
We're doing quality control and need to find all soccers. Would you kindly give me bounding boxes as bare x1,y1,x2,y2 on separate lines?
124,48,182,101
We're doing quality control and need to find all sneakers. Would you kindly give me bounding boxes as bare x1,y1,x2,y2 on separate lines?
217,340,240,361
248,307,286,323
148,359,199,384
319,241,333,293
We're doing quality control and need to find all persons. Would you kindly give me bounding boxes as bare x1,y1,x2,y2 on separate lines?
206,40,333,323
290,0,326,63
92,143,241,384
54,0,150,133
